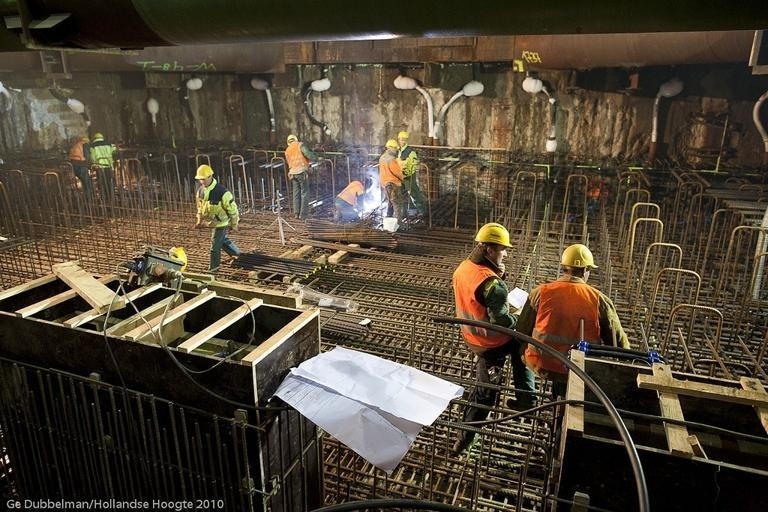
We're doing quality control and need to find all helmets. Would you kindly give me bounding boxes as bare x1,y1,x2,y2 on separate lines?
384,139,400,149
194,164,214,180
80,135,90,142
559,243,599,270
287,134,298,143
91,132,104,144
397,131,410,139
474,222,514,248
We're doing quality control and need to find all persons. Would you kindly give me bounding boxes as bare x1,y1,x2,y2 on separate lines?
451,222,530,457
380,139,410,232
90,133,115,210
69,136,97,201
507,242,632,413
194,164,240,270
393,130,428,218
284,134,325,220
332,177,372,223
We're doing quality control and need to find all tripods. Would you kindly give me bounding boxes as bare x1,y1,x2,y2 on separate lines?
262,190,297,245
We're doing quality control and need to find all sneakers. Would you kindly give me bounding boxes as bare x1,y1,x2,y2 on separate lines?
453,431,481,454
294,213,312,219
506,399,537,416
